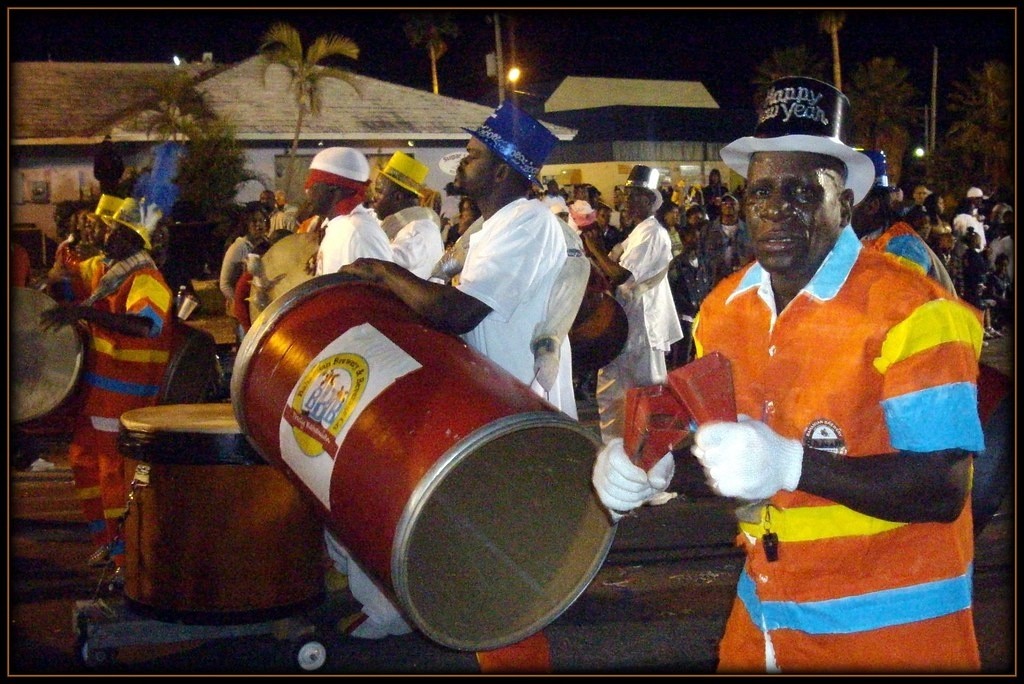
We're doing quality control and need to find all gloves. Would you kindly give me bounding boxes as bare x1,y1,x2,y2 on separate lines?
690,413,804,499
591,434,677,513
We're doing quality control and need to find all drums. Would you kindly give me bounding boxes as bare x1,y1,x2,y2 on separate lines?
249,231,321,325
568,292,628,369
229,272,619,650
9,287,84,432
115,404,326,620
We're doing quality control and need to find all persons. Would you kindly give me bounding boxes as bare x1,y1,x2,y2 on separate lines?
583,165,684,447
337,99,590,672
890,184,1015,346
592,77,984,672
370,152,446,286
305,148,411,640
38,194,197,591
419,188,482,249
219,188,298,334
850,148,956,298
657,168,754,367
530,180,636,250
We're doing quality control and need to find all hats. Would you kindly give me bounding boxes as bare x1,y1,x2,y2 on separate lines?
306,147,369,191
101,197,162,249
858,149,899,193
86,192,124,222
374,150,428,198
720,77,875,205
966,187,984,198
461,100,558,191
618,165,662,214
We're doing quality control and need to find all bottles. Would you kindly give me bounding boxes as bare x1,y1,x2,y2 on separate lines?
176,284,185,311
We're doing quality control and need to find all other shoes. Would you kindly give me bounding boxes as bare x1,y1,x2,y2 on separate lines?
981,327,1003,347
342,605,412,640
30,458,53,472
111,566,126,587
643,491,676,506
83,544,111,568
